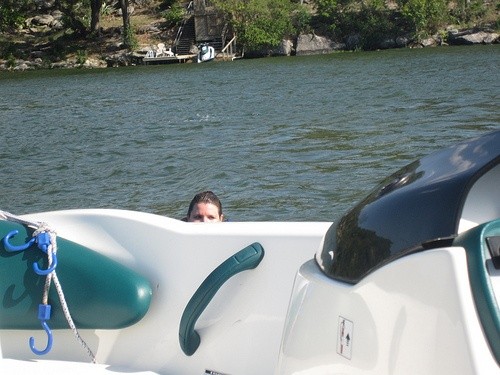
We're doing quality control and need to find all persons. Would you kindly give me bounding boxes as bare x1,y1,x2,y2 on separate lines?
186,191,224,222
